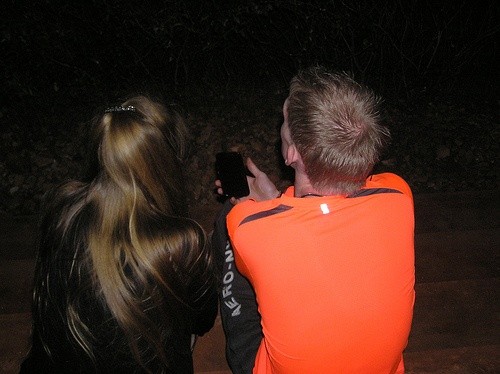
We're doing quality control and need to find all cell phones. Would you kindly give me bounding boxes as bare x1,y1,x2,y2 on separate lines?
217,151,250,199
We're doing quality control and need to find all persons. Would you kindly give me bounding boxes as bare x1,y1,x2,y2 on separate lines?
22,93,217,374
214,70,418,374
